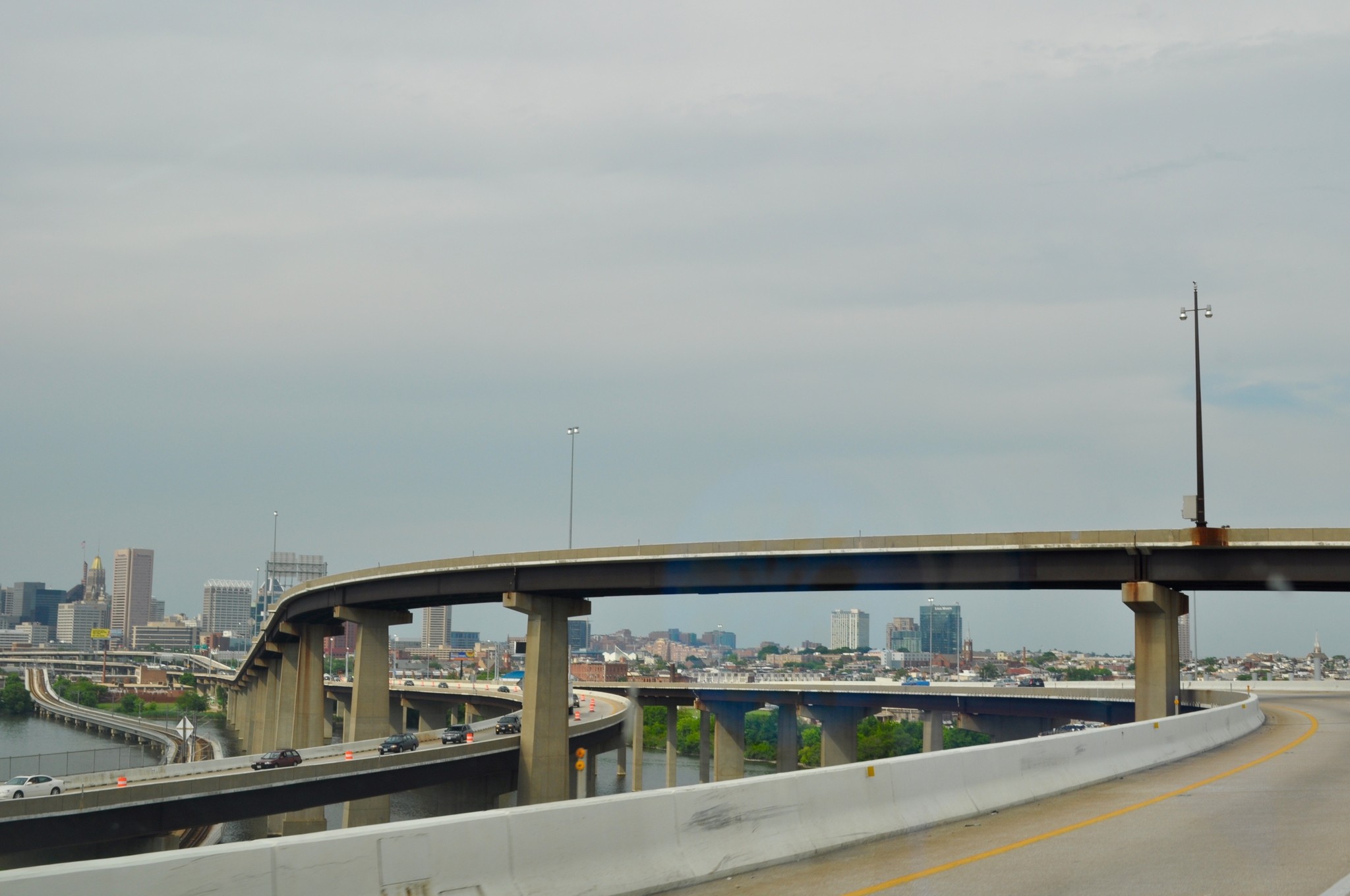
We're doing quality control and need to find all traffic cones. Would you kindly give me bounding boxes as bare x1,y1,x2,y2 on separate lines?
117,776,127,787
466,732,473,744
575,712,580,721
345,750,353,761
588,704,595,713
928,597,935,684
590,699,596,707
580,694,585,702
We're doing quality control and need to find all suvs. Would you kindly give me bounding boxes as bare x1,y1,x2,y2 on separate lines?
251,749,303,770
441,723,473,744
1019,678,1044,688
378,733,420,755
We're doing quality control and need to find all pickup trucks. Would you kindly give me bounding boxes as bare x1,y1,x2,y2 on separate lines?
902,677,930,686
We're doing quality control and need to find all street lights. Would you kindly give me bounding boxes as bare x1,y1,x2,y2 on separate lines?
717,625,723,683
1180,280,1212,527
254,567,259,637
568,426,579,549
272,510,279,603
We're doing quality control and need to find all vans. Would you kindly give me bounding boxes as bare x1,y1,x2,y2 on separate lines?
943,719,954,729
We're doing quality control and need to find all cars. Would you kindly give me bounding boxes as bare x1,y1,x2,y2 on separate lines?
496,715,521,734
323,673,354,683
994,678,1019,688
438,682,448,688
572,693,580,708
405,681,414,686
0,775,66,801
498,686,510,693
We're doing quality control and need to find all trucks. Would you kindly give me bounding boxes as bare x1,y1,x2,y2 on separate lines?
568,681,573,715
